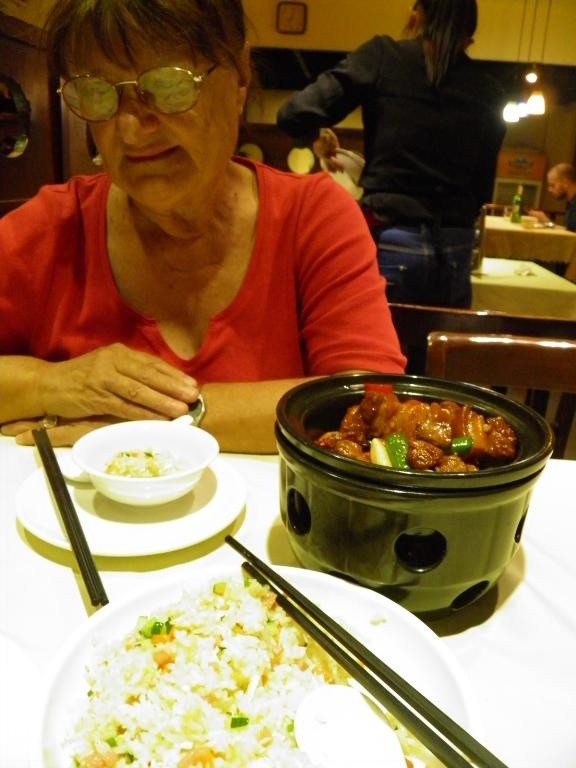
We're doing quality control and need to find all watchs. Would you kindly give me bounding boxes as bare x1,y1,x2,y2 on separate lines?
188,394,206,429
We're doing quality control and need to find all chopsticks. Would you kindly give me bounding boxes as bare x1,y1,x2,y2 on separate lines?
223,533,508,768
31,427,109,606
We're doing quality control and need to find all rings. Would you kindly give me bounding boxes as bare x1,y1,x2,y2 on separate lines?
43,416,58,430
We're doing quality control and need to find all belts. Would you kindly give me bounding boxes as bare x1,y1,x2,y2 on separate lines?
386,214,474,228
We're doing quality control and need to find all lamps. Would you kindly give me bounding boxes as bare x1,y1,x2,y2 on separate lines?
502,0,551,123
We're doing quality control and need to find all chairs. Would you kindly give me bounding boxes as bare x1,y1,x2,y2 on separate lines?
388,302,576,460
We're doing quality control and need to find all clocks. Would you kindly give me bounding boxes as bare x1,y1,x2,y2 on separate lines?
276,2,307,34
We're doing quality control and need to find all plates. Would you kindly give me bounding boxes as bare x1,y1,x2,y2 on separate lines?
14,452,247,558
38,564,483,767
319,148,365,198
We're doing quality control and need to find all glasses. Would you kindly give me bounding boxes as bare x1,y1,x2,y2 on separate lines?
56,54,229,122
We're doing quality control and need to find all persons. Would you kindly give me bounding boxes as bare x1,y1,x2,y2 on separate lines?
238,144,263,162
277,0,506,309
0,0,407,456
527,162,576,282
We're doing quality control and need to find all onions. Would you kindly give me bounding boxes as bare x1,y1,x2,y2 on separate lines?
368,438,390,467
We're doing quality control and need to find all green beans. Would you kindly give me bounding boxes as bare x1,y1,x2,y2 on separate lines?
386,433,472,469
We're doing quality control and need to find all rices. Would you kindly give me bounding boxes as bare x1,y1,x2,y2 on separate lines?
106,450,172,477
73,579,352,768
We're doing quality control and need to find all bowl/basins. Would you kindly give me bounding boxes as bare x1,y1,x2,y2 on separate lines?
273,373,553,492
71,420,220,507
70,419,220,506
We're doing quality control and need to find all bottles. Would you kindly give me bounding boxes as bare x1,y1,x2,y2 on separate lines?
510,184,524,223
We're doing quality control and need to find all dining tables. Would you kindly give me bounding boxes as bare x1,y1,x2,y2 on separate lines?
0,429,576,768
470,258,576,337
484,215,576,281
